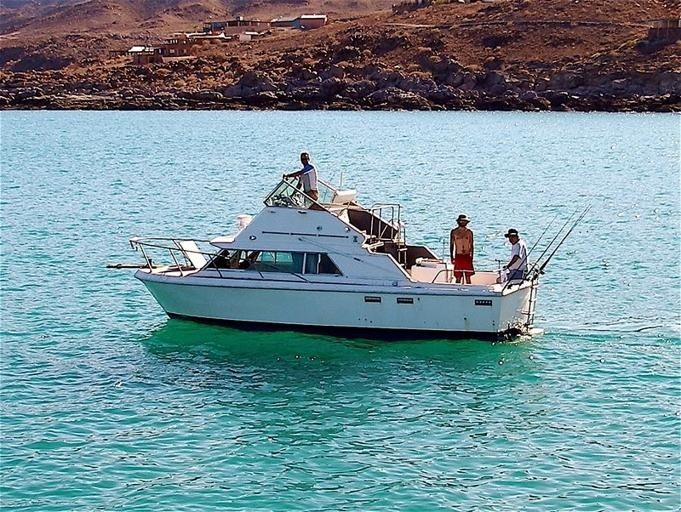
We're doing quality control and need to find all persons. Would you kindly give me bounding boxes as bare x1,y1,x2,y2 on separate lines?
450,213,477,284
283,151,321,211
496,228,529,284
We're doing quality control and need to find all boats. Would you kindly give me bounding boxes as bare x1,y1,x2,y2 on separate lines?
106,176,545,347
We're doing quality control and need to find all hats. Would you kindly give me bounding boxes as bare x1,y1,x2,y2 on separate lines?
456,214,471,223
504,229,518,238
